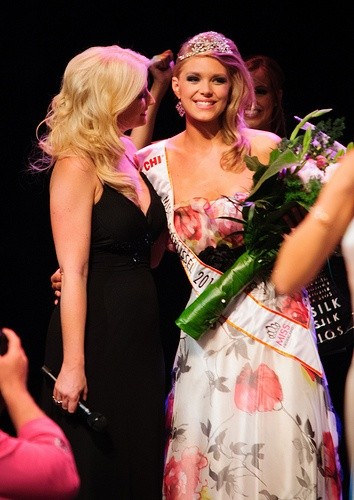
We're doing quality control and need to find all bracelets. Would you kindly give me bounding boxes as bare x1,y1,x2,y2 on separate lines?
308,206,345,236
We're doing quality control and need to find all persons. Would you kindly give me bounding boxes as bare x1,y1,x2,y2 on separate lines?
0,328,80,500
273,149,354,500
128,50,285,152
51,31,342,500
29,45,169,500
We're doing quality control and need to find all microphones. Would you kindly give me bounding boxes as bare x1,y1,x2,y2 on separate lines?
41,364,109,433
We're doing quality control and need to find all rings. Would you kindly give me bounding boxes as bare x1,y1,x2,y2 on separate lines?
53,397,62,407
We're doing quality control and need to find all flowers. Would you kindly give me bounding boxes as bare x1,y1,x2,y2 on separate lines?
176,108,354,340
